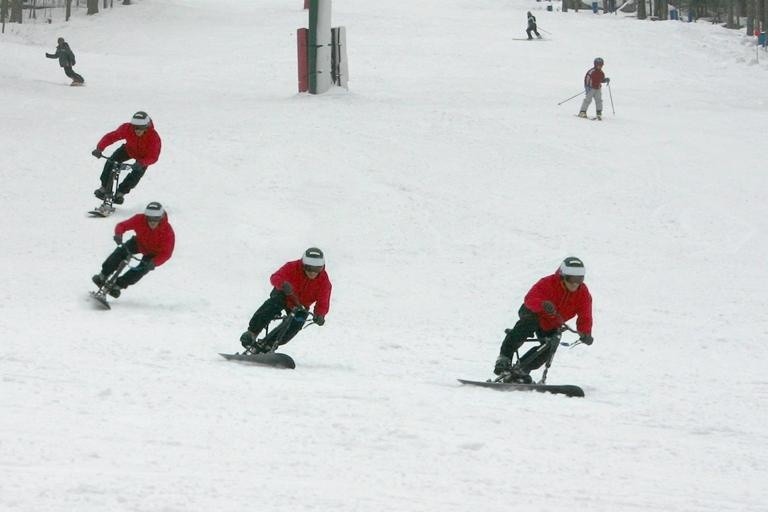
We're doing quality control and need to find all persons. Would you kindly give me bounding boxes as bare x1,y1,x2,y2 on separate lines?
92,202,175,298
494,257,594,383
526,11,541,40
92,111,161,204
578,57,610,120
240,248,332,355
45,37,85,86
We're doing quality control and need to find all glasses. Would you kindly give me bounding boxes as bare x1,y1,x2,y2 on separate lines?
148,217,161,221
305,265,322,272
565,275,584,283
134,125,148,129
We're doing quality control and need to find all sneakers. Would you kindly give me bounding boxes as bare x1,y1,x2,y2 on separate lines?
596,113,601,119
96,186,106,196
111,283,122,297
579,111,587,117
241,331,256,343
495,359,506,375
512,364,532,384
115,192,123,203
93,272,106,283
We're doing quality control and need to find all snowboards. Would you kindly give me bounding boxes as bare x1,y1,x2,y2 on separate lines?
89,207,115,218
72,79,84,86
92,295,111,310
219,353,295,369
458,378,584,397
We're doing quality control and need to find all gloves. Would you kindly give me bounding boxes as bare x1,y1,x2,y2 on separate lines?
114,234,122,244
544,302,554,313
312,313,325,326
580,335,593,345
132,163,142,172
144,261,154,270
92,149,102,158
283,281,293,295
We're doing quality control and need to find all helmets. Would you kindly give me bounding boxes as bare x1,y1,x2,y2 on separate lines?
302,248,325,267
560,258,585,276
594,58,603,66
144,202,163,217
130,112,150,126
